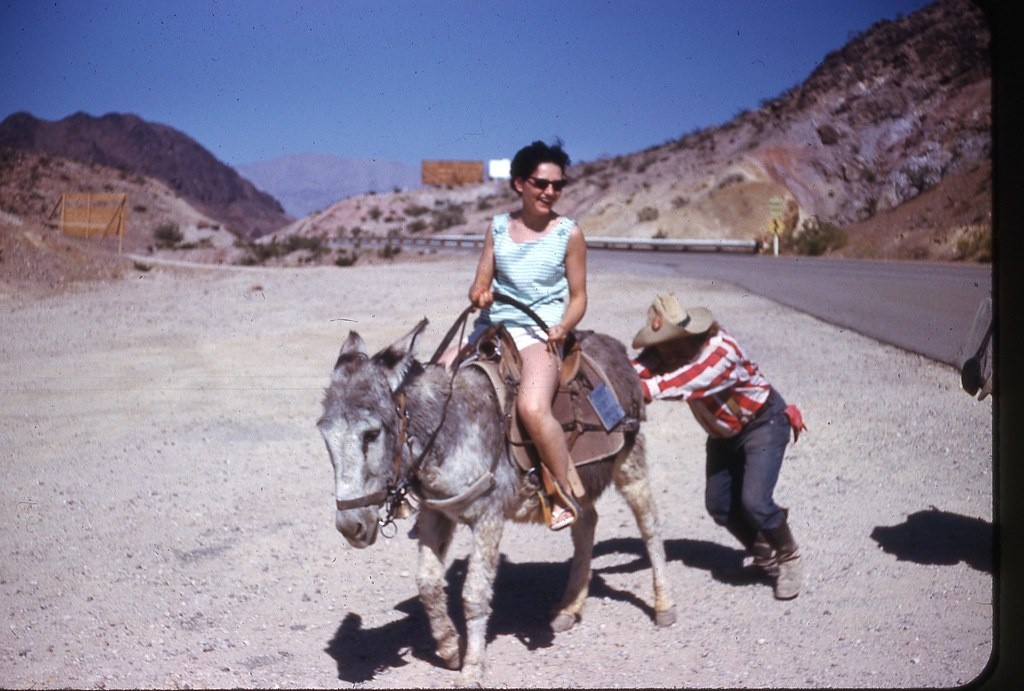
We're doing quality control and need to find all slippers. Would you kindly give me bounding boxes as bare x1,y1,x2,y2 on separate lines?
550,507,577,530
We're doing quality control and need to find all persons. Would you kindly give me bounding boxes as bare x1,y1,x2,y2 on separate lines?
631,292,806,600
469,135,589,531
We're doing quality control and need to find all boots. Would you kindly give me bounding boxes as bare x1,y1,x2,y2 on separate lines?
717,515,771,578
762,508,803,599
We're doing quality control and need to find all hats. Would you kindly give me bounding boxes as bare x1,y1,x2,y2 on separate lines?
632,293,713,349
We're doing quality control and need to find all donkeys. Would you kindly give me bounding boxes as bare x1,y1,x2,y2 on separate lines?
315,318,676,689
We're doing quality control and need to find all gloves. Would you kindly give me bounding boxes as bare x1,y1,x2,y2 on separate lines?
784,405,808,444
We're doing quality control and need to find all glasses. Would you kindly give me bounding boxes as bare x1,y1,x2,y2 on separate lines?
527,176,568,191
